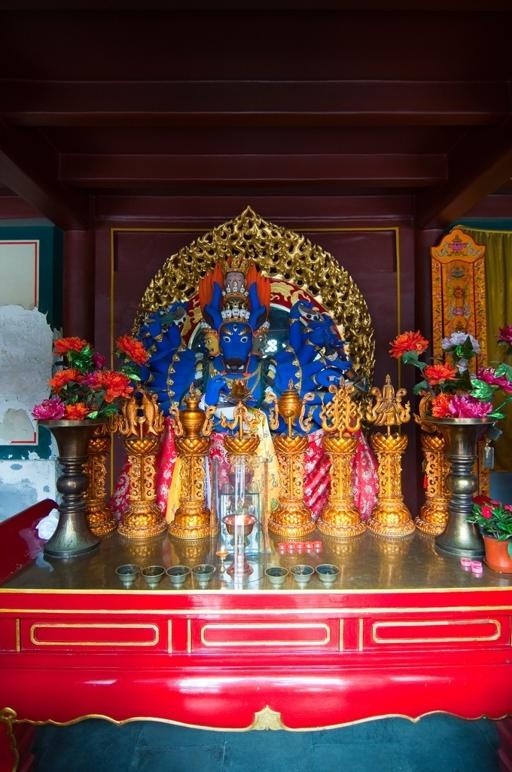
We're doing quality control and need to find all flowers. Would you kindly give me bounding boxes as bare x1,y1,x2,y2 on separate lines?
388,325,512,418
32,334,151,420
465,494,512,541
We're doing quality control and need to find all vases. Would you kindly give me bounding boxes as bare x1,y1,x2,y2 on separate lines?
479,533,511,573
423,416,495,557
37,418,110,560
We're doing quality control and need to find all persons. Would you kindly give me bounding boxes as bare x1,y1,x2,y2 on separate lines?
222,256,252,292
